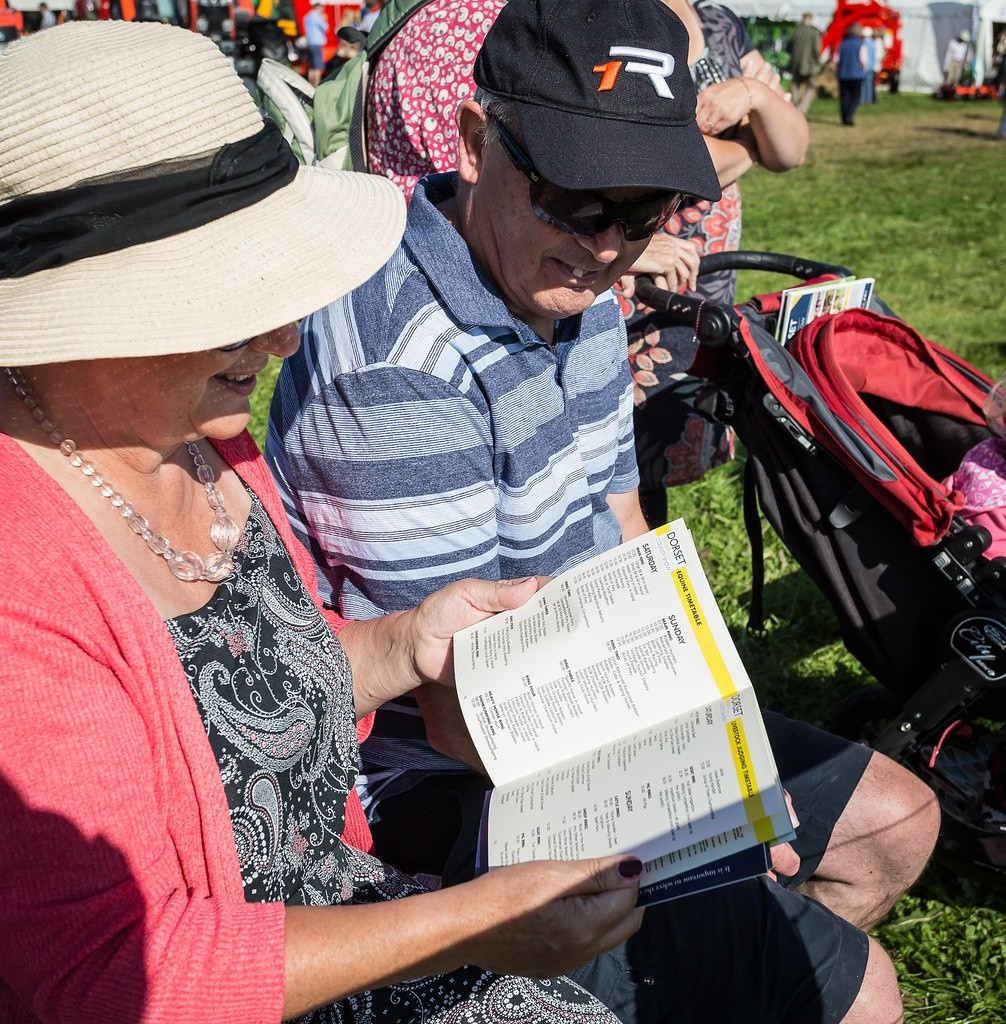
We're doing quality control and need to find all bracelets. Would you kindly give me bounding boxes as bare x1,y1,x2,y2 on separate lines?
735,76,753,110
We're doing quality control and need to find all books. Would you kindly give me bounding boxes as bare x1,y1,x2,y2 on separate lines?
453,517,798,908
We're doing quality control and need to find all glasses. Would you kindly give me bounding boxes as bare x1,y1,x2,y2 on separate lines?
215,337,257,352
496,121,686,243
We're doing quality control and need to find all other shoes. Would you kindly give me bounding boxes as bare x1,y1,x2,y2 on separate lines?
842,119,856,130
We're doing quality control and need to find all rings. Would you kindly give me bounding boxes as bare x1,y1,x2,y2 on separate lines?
707,118,714,127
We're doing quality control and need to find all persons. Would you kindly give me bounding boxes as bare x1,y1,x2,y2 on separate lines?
833,24,868,126
786,11,823,114
943,30,971,86
321,0,810,531
871,29,884,103
304,3,329,87
940,377,1006,564
859,26,875,105
40,2,56,29
0,19,645,1024
264,0,941,1022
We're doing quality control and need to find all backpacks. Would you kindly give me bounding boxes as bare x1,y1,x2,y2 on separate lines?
254,0,430,171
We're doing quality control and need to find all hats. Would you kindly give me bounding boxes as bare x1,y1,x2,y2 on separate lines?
472,1,722,203
0,19,409,368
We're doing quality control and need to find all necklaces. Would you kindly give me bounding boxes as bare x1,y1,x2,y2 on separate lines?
4,366,240,582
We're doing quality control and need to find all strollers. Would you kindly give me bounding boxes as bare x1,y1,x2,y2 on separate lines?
630,246,1004,912
